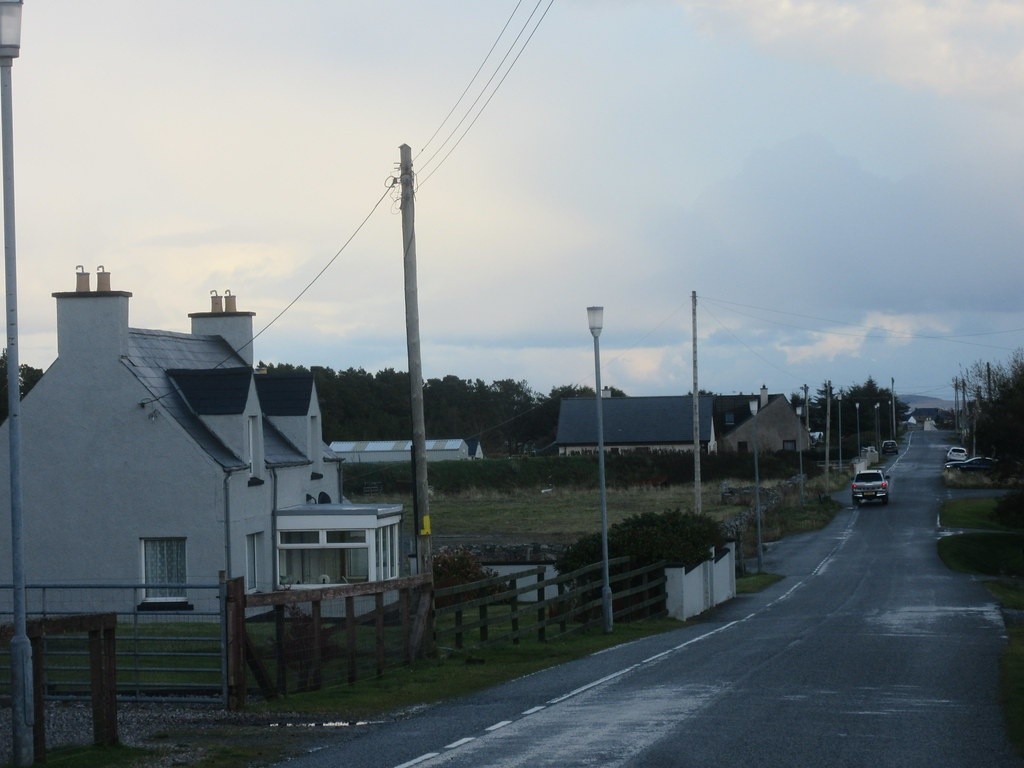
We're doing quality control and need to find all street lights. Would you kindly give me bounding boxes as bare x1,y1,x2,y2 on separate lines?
855,402,862,462
0,1,45,768
876,403,882,448
874,405,880,451
887,401,893,440
583,304,620,636
836,391,844,470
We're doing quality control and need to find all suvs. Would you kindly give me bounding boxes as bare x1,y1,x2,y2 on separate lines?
852,469,891,506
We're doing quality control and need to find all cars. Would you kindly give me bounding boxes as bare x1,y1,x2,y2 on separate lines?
881,440,898,455
947,447,968,461
944,456,999,472
